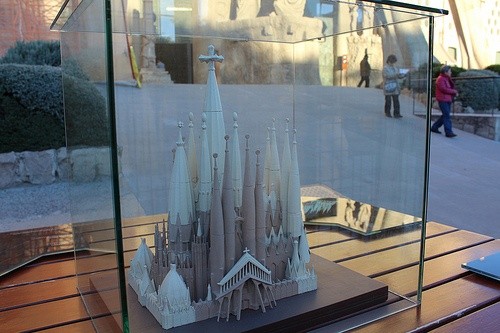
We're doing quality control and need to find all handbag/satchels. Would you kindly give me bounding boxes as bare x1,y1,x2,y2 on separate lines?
384,82,397,92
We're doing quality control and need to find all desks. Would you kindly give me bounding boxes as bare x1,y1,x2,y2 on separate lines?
0,196,500,333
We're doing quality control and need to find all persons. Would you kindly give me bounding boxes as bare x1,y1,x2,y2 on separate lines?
383,55,403,118
432,66,459,137
358,56,370,88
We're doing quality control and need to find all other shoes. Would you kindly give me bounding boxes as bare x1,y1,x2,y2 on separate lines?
431,129,442,134
449,133,458,137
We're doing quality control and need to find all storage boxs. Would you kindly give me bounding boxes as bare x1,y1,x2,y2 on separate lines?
50,0,449,333
